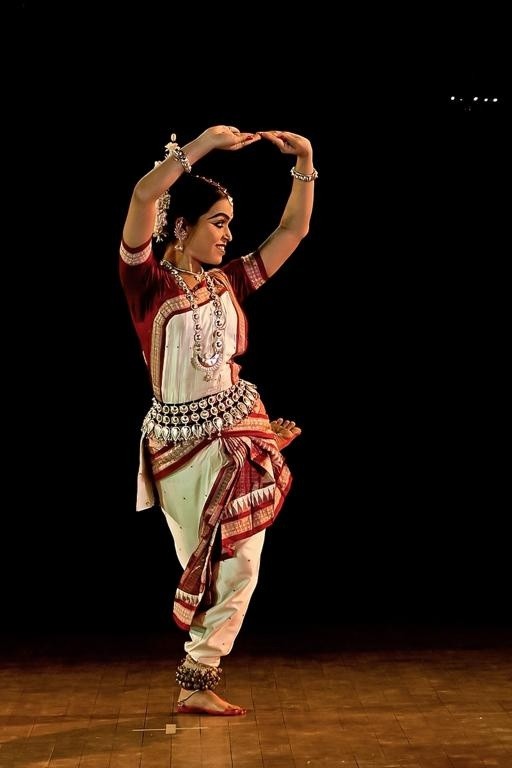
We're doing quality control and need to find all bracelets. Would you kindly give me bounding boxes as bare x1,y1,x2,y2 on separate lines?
170,146,193,175
290,165,319,182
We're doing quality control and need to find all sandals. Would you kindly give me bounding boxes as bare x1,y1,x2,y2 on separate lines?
174,683,246,718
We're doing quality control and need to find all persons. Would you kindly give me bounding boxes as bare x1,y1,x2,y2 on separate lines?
121,123,321,715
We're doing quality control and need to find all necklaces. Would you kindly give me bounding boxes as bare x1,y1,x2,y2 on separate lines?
174,267,204,284
159,260,224,382
